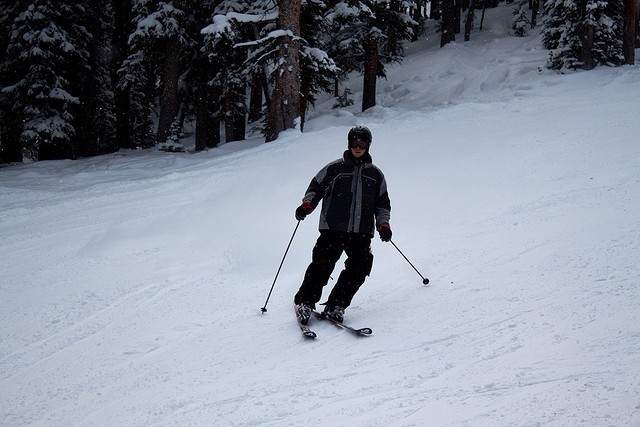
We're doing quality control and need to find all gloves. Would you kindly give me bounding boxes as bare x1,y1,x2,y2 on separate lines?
376,224,392,242
296,201,314,220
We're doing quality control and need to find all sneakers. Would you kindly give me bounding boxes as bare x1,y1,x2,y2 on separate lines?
296,302,311,324
324,305,344,322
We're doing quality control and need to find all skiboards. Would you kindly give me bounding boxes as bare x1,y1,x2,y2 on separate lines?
296,310,372,337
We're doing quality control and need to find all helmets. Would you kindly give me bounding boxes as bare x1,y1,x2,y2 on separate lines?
348,126,372,143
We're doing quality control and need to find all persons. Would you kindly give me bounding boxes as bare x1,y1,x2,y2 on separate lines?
295,126,392,325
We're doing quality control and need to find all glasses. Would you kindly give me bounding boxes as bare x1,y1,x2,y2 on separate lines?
349,139,371,150
